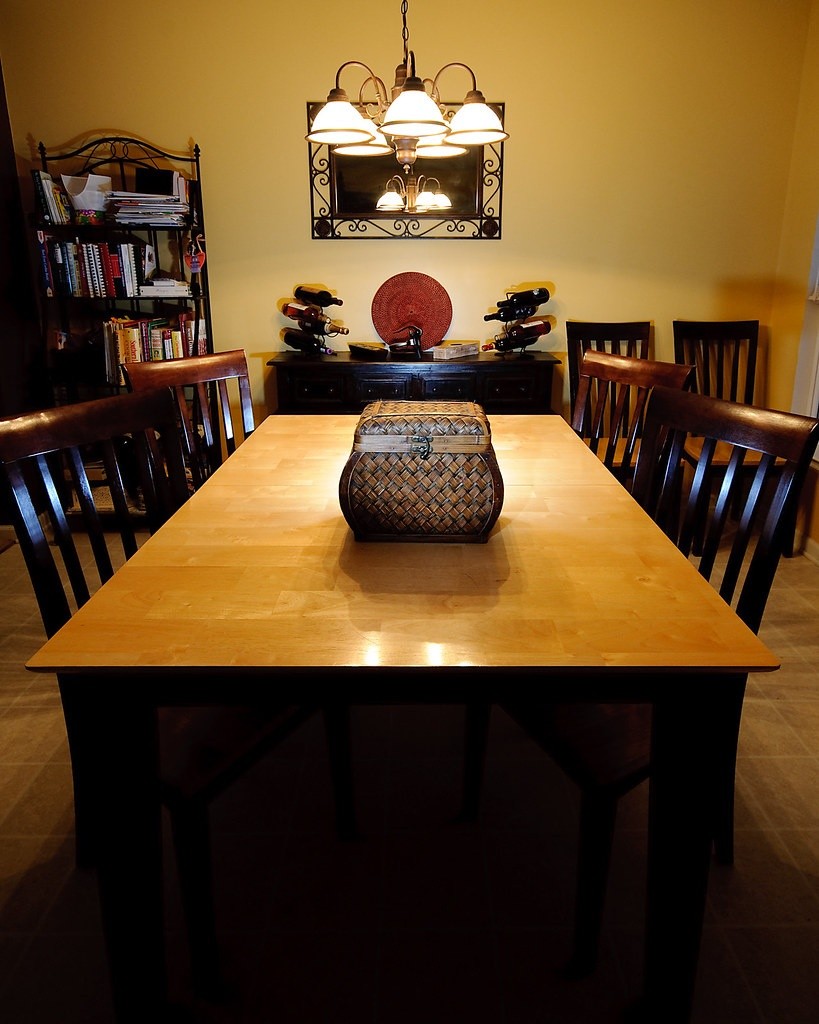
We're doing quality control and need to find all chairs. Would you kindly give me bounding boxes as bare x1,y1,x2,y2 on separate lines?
0,381,186,645
568,347,697,516
450,395,818,974
117,348,259,516
565,320,684,545
670,318,804,558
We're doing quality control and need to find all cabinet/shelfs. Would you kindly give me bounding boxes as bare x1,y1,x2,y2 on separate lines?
30,133,215,472
265,350,562,415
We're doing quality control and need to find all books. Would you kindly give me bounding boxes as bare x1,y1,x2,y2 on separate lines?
30,168,75,225
103,305,207,387
36,230,194,298
104,166,198,227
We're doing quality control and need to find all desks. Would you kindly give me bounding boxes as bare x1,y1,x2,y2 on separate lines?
24,411,783,1014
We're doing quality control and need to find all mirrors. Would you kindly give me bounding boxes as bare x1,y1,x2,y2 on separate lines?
305,98,504,243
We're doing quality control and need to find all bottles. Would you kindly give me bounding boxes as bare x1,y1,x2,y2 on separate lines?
484,288,550,322
279,327,332,354
482,320,551,352
282,286,349,335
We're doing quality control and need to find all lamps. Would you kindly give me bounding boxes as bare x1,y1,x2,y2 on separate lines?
375,161,453,214
304,0,511,173
